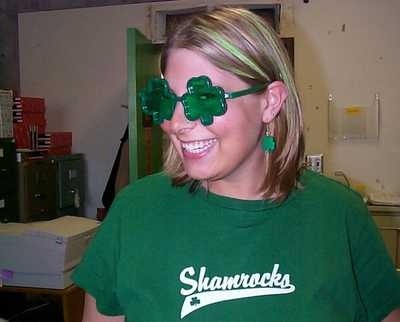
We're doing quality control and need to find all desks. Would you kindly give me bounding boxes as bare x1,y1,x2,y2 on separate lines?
0,284,86,322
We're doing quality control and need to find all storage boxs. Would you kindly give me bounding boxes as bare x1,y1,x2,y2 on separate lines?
0,88,88,224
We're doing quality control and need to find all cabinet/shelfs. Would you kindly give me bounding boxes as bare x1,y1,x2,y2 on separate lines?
366,205,399,276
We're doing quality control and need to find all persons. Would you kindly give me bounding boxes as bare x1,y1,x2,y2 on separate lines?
70,4,400,322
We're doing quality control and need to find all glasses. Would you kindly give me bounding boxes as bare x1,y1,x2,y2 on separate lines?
140,74,268,127
